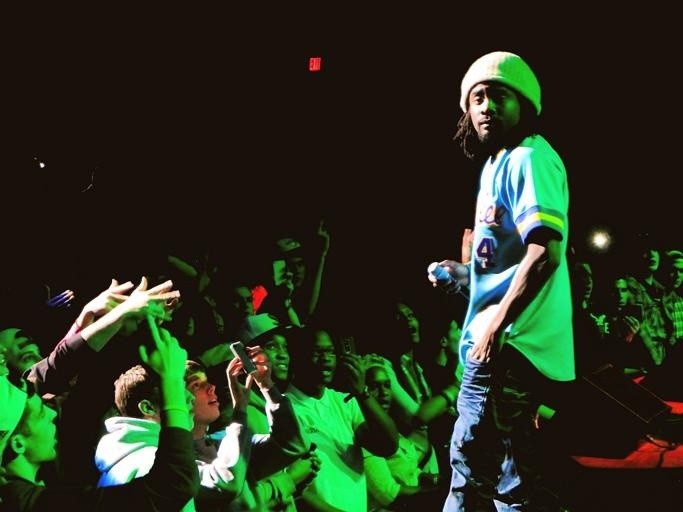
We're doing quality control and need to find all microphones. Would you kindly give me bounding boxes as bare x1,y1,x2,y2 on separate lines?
426,262,470,302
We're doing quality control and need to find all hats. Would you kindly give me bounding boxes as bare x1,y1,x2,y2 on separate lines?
1,328,39,466
240,313,292,348
459,51,541,116
665,249,683,261
569,260,592,278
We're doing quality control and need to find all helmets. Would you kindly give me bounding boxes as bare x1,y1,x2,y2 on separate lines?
265,237,303,267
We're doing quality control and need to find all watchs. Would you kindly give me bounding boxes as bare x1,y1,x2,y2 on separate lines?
344,386,369,403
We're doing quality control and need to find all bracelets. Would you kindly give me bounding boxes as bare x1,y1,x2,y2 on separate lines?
162,405,188,413
443,385,460,406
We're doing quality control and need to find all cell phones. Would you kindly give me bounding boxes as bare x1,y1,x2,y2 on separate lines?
617,306,643,322
272,260,287,287
231,342,257,375
341,338,352,356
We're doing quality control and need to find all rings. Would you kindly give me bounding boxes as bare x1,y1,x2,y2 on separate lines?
634,324,636,326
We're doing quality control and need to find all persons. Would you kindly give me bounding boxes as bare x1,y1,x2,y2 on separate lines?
0,219,683,512
427,50,576,512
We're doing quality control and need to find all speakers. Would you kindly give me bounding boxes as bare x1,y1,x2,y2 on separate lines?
577,363,672,459
